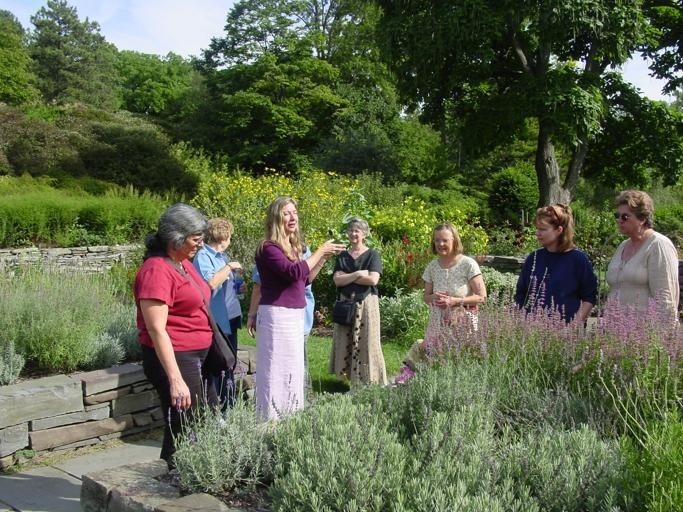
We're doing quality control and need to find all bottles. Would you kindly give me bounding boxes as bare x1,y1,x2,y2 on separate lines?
234,268,246,301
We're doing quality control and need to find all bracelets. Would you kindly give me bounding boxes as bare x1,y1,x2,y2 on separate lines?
245,313,257,321
225,263,232,270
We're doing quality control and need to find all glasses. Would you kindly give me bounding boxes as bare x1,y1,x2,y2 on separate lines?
614,213,632,222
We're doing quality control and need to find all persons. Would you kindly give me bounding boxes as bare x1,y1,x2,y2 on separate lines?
605,188,680,338
254,195,349,430
420,222,487,371
514,201,598,339
131,201,234,474
191,216,247,416
245,238,316,405
329,217,390,389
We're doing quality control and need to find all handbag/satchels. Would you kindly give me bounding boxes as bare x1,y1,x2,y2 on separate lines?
331,300,357,326
207,321,238,377
